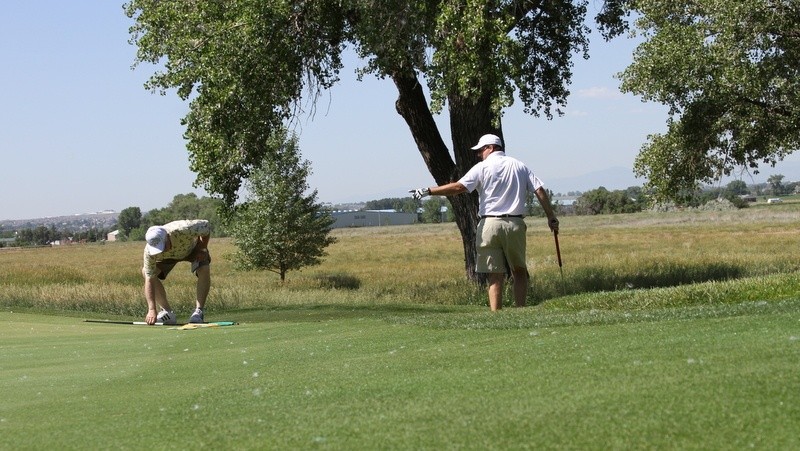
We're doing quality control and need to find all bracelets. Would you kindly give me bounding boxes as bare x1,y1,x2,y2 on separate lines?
428,188,432,195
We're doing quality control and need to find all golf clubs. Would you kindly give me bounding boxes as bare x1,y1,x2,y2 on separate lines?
551,219,567,296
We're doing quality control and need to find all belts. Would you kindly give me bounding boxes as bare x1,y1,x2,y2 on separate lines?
481,214,523,217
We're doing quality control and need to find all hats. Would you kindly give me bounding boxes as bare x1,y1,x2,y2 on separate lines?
471,134,503,150
145,226,168,256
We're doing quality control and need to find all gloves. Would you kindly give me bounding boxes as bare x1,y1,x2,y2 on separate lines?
408,188,431,200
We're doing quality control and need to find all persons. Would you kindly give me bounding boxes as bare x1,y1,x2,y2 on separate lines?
140,219,211,324
409,133,558,312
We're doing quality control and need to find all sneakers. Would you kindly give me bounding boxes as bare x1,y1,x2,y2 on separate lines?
156,311,177,324
189,309,204,322
